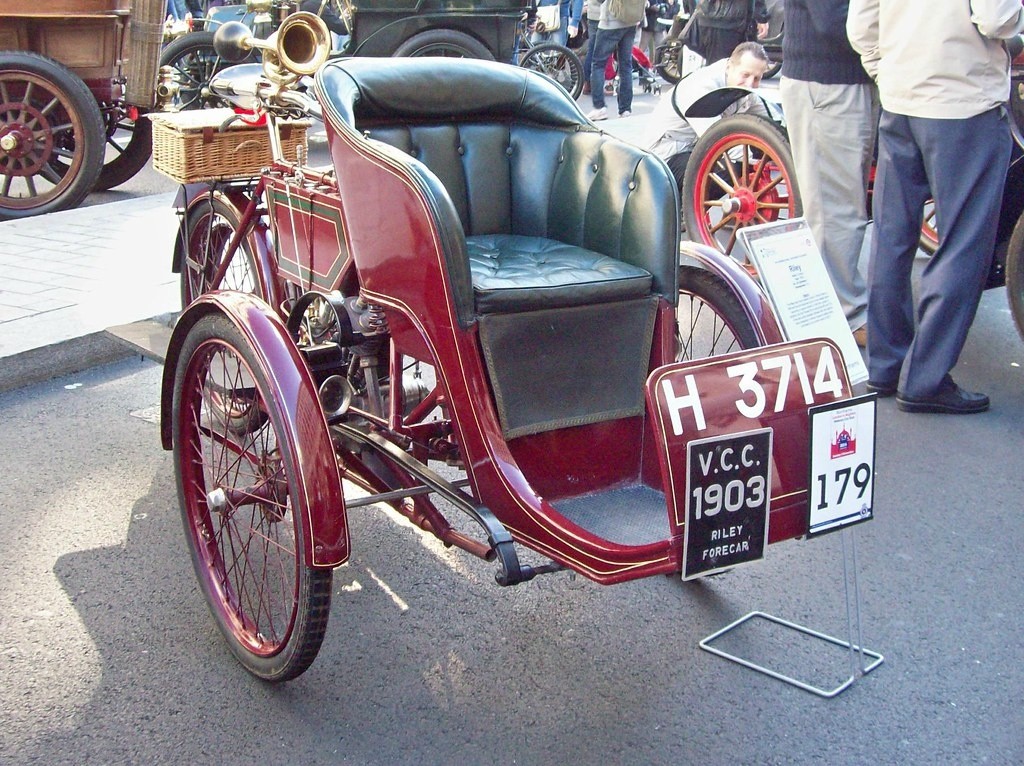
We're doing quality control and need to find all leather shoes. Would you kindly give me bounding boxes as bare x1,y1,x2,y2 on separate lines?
866,380,899,399
896,384,991,414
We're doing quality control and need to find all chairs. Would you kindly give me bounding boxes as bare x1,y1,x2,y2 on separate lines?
309,53,686,431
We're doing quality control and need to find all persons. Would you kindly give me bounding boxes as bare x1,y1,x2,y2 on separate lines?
582,0,769,121
643,40,786,208
300,0,348,51
531,0,583,63
782,0,880,348
845,0,1024,416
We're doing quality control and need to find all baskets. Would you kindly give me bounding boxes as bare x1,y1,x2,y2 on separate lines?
147,104,314,184
125,0,166,107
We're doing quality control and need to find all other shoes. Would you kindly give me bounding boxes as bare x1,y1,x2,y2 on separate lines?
582,80,591,95
585,105,608,121
617,110,632,119
603,88,614,96
851,322,867,347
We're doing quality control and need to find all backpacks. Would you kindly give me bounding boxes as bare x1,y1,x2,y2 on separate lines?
608,0,647,25
567,18,588,49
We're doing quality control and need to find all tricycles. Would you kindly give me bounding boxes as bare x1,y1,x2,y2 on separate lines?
1,0,1024,331
156,12,879,684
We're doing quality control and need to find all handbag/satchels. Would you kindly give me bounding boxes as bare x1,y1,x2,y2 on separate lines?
677,8,707,60
532,5,561,33
